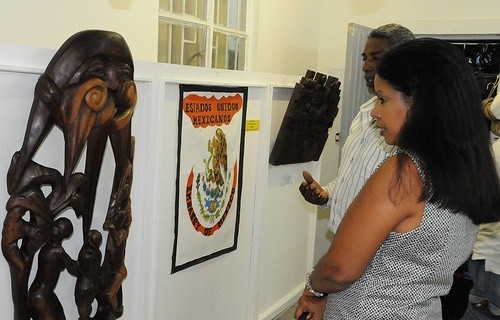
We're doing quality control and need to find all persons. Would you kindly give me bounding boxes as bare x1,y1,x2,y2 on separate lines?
294,38,500,320
299,22,416,236
459,73,500,319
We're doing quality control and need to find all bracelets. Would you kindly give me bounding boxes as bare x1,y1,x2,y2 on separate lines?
305,271,328,298
